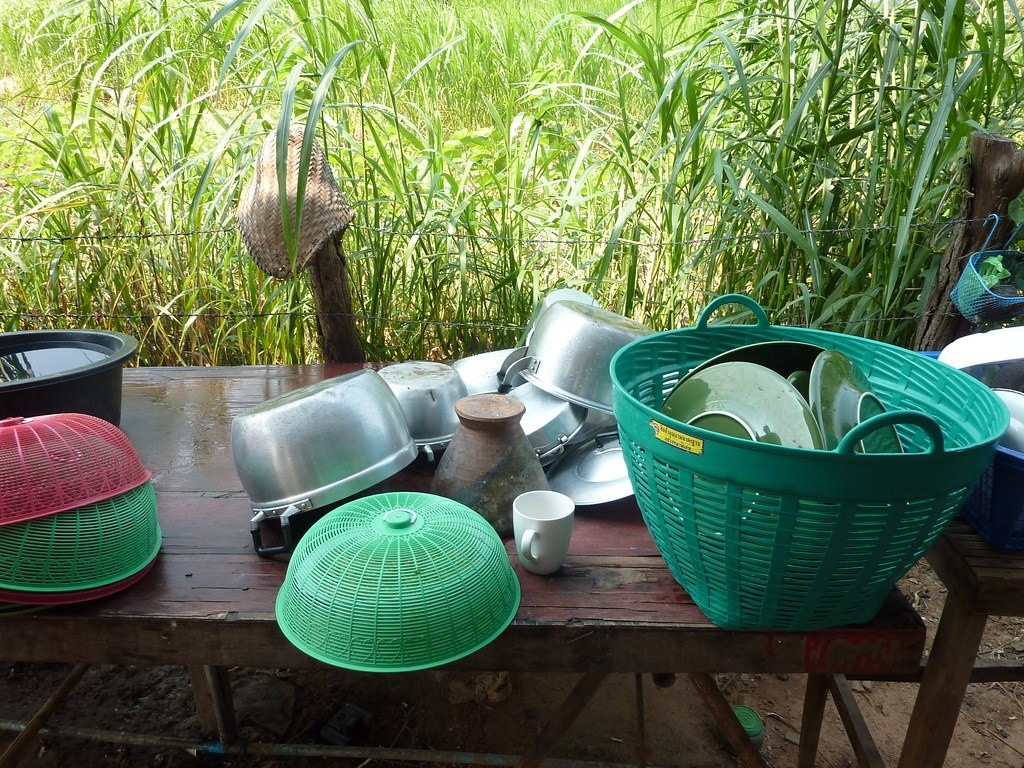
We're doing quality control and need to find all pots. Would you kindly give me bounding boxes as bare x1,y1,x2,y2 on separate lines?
378,362,468,460
452,286,661,505
235,368,418,557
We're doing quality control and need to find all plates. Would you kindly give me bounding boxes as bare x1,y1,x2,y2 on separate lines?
663,341,905,588
938,325,1024,453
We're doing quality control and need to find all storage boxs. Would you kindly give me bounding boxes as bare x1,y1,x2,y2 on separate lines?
919,351,1024,552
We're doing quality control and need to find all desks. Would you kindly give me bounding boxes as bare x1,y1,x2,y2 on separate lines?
0,365,926,768
797,510,1024,768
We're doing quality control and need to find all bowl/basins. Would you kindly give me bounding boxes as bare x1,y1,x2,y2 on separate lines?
0,330,139,428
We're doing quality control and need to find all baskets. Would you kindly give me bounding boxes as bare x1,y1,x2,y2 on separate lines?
275,491,523,674
604,293,1011,633
912,349,1024,549
950,247,1024,324
0,412,153,526
0,427,163,606
233,124,355,280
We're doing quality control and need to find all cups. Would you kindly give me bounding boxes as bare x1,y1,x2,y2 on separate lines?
514,490,576,575
